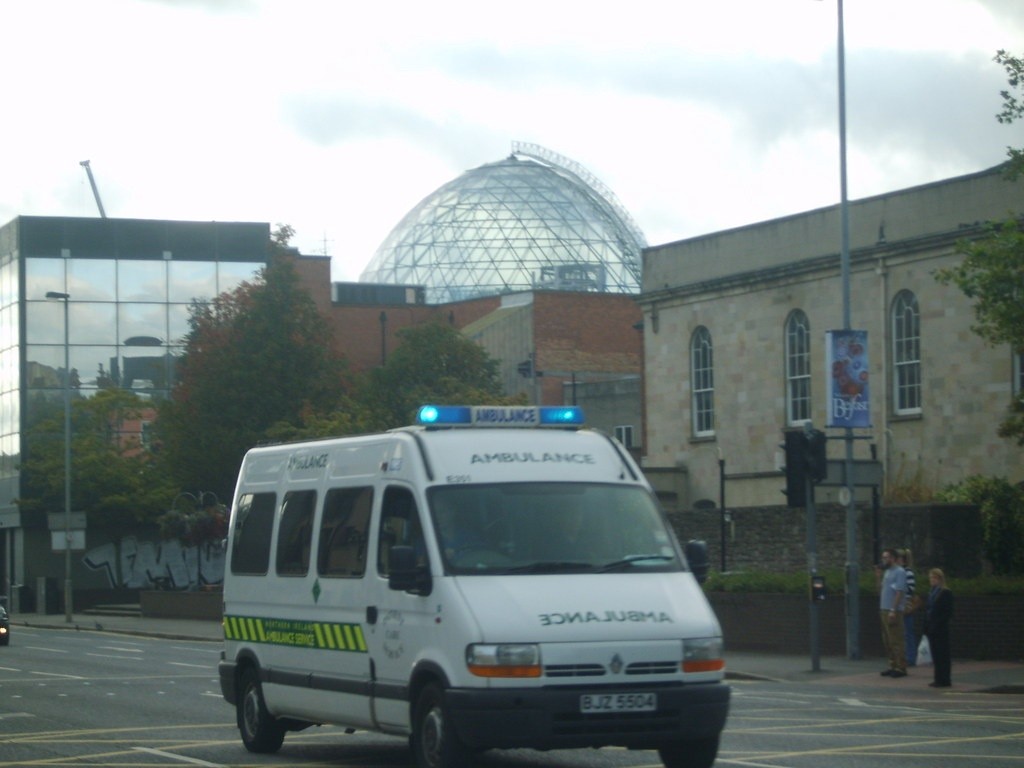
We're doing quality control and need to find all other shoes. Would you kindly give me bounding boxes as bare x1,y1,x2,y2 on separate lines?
929,681,951,687
890,671,907,678
881,669,893,676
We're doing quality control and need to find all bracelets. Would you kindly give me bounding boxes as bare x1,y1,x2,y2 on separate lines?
889,608,895,611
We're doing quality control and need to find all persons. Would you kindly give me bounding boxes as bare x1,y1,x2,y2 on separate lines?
922,568,955,688
879,548,917,678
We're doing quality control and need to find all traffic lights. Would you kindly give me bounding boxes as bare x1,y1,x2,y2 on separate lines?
809,574,830,603
776,426,815,507
799,429,827,478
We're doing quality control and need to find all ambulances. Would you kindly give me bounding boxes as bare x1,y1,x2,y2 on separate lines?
217,403,734,767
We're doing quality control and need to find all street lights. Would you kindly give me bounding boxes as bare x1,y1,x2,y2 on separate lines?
43,291,75,623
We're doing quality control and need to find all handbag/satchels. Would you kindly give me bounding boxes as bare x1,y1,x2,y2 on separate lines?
916,635,933,666
903,593,922,614
